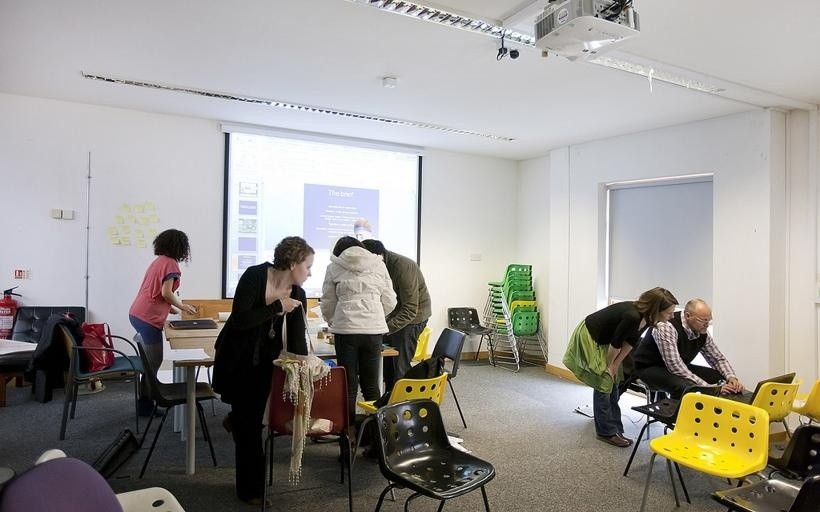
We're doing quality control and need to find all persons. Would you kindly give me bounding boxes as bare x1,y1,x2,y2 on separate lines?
320,236,398,461
129,227,196,419
564,287,678,447
634,298,746,401
208,236,315,509
361,241,432,394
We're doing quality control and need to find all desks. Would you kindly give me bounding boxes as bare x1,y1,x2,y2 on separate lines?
164,319,397,474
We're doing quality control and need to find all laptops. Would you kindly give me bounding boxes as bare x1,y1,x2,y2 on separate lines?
722,372,795,404
169,319,219,329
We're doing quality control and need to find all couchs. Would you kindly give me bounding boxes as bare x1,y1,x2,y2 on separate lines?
3,306,86,392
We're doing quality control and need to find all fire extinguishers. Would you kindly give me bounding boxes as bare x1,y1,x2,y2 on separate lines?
0,285,22,339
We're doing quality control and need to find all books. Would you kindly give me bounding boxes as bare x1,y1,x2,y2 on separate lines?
169,318,217,330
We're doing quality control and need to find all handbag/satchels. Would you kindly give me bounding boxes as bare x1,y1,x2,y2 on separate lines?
261,303,334,433
373,353,444,409
80,321,114,372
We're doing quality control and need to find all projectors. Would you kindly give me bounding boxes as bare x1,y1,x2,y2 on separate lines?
533,0,641,58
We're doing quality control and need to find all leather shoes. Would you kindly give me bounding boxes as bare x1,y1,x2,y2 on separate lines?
596,433,632,446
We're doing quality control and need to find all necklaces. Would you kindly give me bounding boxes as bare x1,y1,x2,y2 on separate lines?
269,313,280,339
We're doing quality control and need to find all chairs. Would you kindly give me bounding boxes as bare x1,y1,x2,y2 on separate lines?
623,379,820,512
415,327,468,427
376,399,495,509
4,458,122,512
409,327,432,364
351,372,448,471
37,449,183,512
133,333,219,477
448,308,495,365
483,263,548,373
266,366,348,485
59,324,145,439
